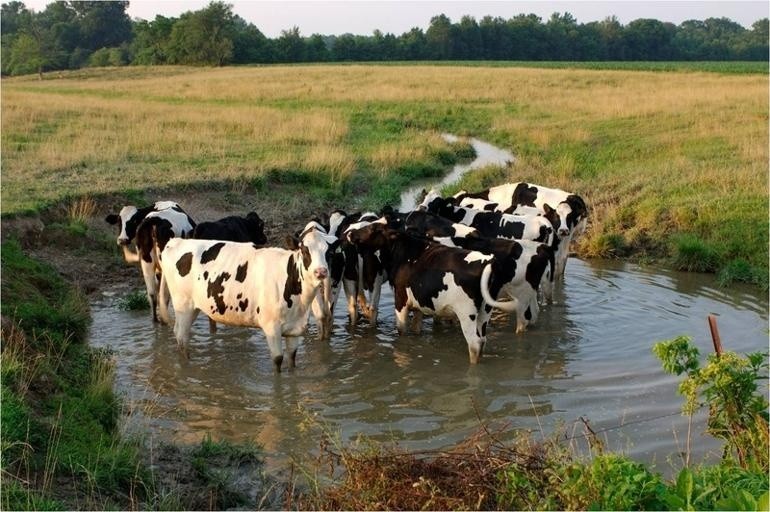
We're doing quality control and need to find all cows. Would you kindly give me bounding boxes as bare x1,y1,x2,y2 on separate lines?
157,227,340,374
295,182,587,366
105,201,196,324
187,212,267,332
